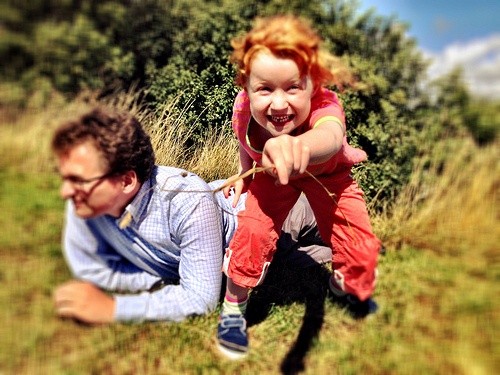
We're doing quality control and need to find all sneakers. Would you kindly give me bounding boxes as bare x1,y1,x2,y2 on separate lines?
215,311,248,360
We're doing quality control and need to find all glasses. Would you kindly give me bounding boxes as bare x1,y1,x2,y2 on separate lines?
64,172,117,190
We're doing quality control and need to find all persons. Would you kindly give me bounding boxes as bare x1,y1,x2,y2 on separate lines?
214,14,382,362
52,109,332,324
